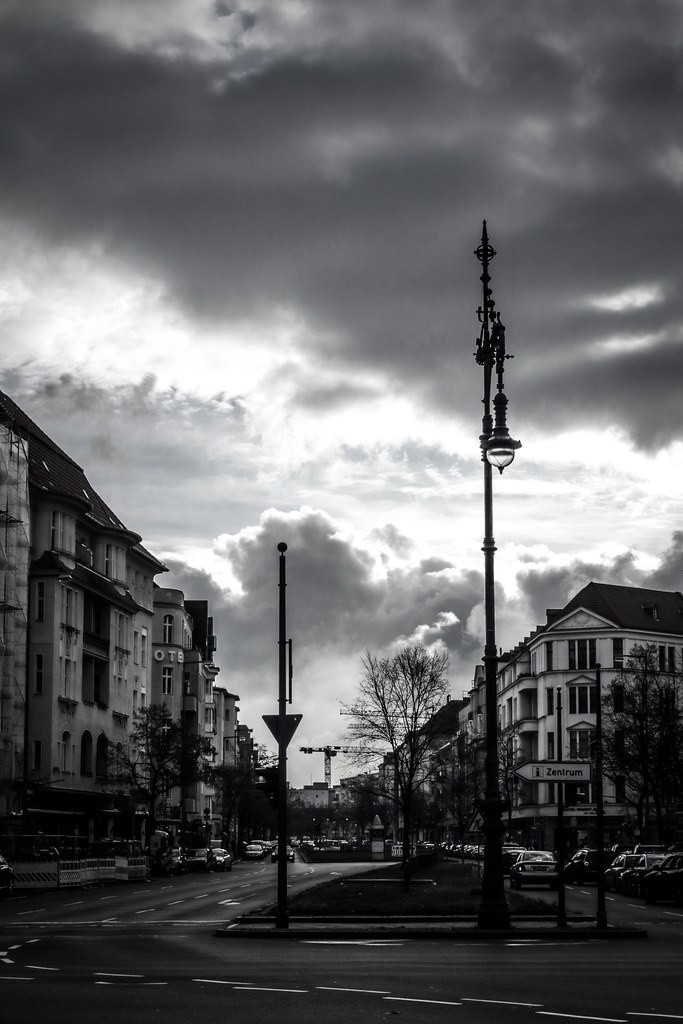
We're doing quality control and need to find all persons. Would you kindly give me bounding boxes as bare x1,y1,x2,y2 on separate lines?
577,837,584,849
528,844,534,850
223,832,230,850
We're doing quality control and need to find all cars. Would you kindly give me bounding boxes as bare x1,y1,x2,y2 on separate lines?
563,847,614,883
0,854,16,897
609,842,683,903
209,847,232,872
249,838,280,851
245,844,266,861
290,836,528,865
272,845,296,863
185,849,211,869
508,850,559,888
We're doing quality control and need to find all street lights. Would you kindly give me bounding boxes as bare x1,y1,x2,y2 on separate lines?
222,735,246,837
476,218,524,934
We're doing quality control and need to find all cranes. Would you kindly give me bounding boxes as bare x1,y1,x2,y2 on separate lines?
300,745,388,788
